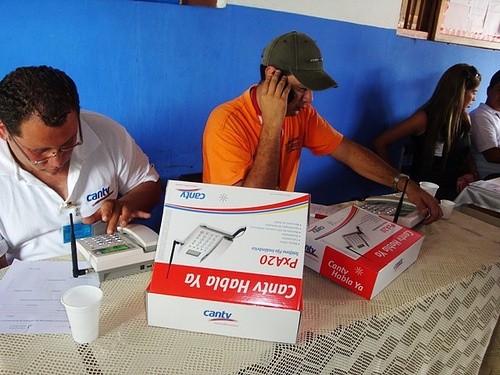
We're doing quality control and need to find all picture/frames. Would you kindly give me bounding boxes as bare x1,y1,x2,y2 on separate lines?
434,0,500,49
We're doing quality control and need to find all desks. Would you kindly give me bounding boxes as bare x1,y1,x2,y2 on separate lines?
0,187,500,375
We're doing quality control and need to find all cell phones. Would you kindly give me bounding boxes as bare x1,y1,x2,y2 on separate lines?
271,71,296,104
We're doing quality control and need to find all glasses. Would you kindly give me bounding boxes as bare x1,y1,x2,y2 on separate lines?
4,115,85,167
473,73,483,81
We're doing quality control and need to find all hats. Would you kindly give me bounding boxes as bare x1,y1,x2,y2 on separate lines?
261,31,339,92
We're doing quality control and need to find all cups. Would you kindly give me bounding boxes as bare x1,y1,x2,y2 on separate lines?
440,200,455,220
419,182,439,197
61,285,103,344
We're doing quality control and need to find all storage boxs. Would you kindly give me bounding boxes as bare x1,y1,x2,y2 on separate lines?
304,203,425,299
146,179,311,346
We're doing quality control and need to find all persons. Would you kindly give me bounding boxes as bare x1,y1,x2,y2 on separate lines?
373,63,482,197
0,65,164,269
202,31,444,225
469,70,500,180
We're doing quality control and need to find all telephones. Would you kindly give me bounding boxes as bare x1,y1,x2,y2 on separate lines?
357,177,431,230
340,231,373,264
164,225,234,280
68,212,161,281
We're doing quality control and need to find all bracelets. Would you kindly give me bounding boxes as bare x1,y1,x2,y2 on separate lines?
393,174,410,192
